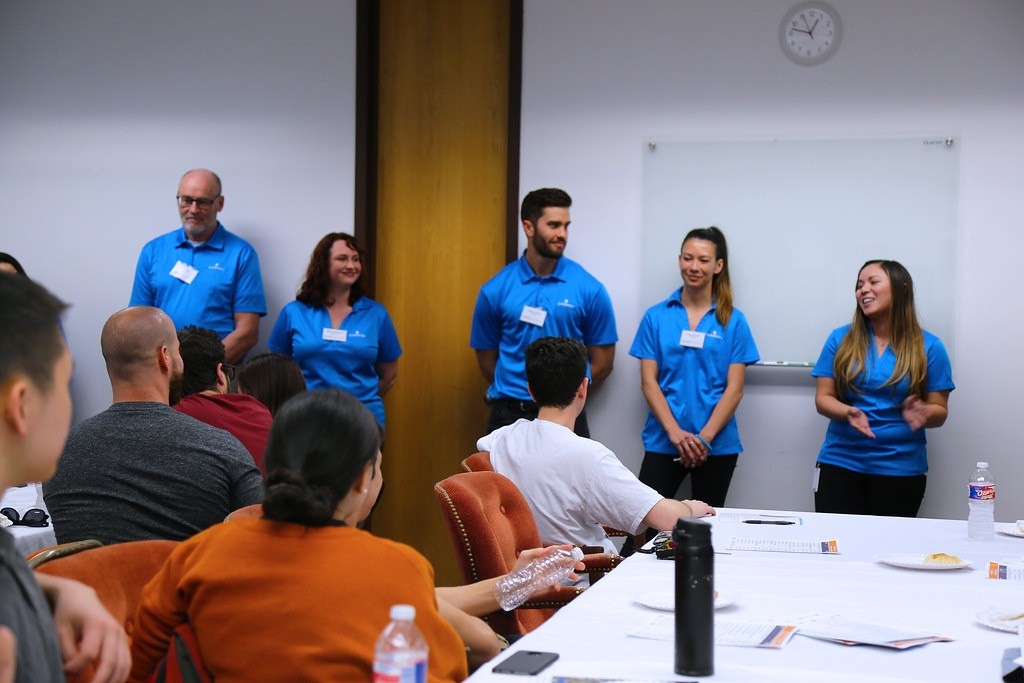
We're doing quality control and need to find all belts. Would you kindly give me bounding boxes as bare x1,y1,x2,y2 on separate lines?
499,397,539,413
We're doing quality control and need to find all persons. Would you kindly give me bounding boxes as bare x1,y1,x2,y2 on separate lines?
130,386,468,683
129,169,268,360
267,233,403,447
42,305,263,547
469,188,618,440
168,324,274,485
475,336,717,590
0,268,133,683
617,226,760,559
0,250,71,351
223,419,586,660
810,260,956,518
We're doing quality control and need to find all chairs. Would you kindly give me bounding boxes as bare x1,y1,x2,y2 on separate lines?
434,451,623,645
24,539,183,683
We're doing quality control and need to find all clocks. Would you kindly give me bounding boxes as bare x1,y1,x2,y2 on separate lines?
779,1,844,67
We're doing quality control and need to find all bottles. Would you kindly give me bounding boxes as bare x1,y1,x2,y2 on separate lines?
493,547,585,612
967,461,994,542
672,517,715,676
373,604,429,683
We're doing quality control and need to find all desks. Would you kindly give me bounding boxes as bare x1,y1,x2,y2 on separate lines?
452,507,1024,683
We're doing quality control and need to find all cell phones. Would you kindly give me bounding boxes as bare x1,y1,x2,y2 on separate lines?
493,651,558,675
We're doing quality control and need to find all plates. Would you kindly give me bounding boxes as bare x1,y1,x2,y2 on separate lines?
632,585,729,612
971,600,1024,633
873,553,972,569
995,522,1024,537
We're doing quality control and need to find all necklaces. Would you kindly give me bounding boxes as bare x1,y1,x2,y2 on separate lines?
875,334,888,347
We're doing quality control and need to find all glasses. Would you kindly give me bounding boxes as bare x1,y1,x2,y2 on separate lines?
0,508,49,527
222,364,235,381
176,191,221,210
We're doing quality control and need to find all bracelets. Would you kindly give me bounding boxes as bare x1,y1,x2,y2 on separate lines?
680,501,693,519
696,434,712,456
